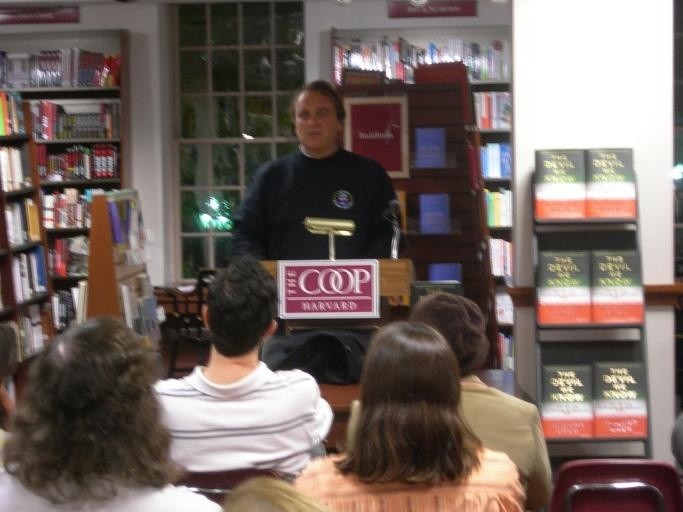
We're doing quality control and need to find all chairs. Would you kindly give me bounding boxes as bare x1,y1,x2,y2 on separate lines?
546,455,683,511
174,468,277,506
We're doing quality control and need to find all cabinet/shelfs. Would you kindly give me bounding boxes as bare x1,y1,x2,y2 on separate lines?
531,167,653,459
336,80,507,374
1,27,132,339
84,186,148,323
1,102,54,433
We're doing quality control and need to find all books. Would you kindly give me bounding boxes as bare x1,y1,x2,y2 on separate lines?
410,262,462,311
534,150,648,439
336,34,515,84
416,128,445,167
0,45,160,414
475,91,516,361
342,98,410,232
419,194,450,233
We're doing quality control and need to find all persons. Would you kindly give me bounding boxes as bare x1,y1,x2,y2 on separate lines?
221,474,322,512
226,79,407,261
292,321,530,511
138,258,333,478
401,285,555,510
0,312,224,512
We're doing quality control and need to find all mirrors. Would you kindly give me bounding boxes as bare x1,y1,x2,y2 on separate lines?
331,22,516,378
